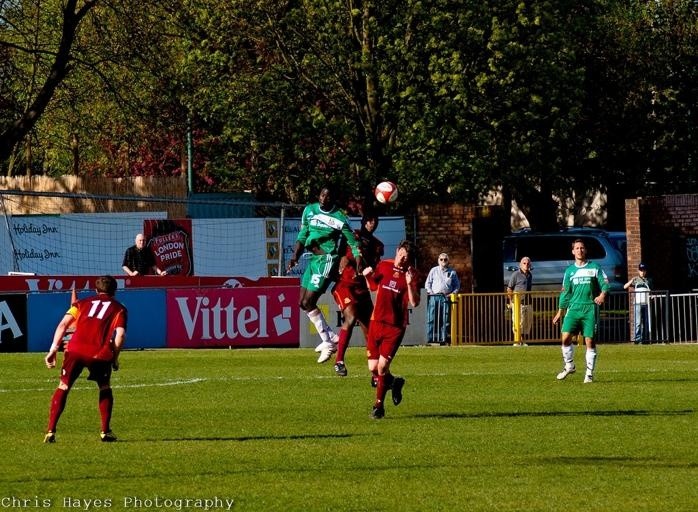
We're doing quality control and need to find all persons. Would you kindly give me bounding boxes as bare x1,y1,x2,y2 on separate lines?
506,256,534,346
285,186,364,364
623,263,654,345
121,233,167,277
329,209,385,388
43,275,129,443
360,239,421,421
424,251,461,346
550,238,611,384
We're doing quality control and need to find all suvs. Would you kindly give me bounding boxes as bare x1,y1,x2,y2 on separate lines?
503,231,626,295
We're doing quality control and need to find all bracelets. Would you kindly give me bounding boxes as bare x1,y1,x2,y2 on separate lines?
49,343,60,355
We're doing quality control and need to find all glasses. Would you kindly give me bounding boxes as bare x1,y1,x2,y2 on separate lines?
440,259,447,262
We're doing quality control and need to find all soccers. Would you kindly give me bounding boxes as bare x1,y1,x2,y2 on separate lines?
375,182,398,204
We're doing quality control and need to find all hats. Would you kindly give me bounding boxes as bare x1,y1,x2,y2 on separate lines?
639,263,646,270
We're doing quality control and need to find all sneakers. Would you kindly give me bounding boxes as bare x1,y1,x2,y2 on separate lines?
556,364,576,380
373,402,384,419
317,342,337,363
334,361,347,376
392,377,405,406
584,374,592,383
372,376,378,387
44,432,55,444
100,430,117,442
315,334,339,353
